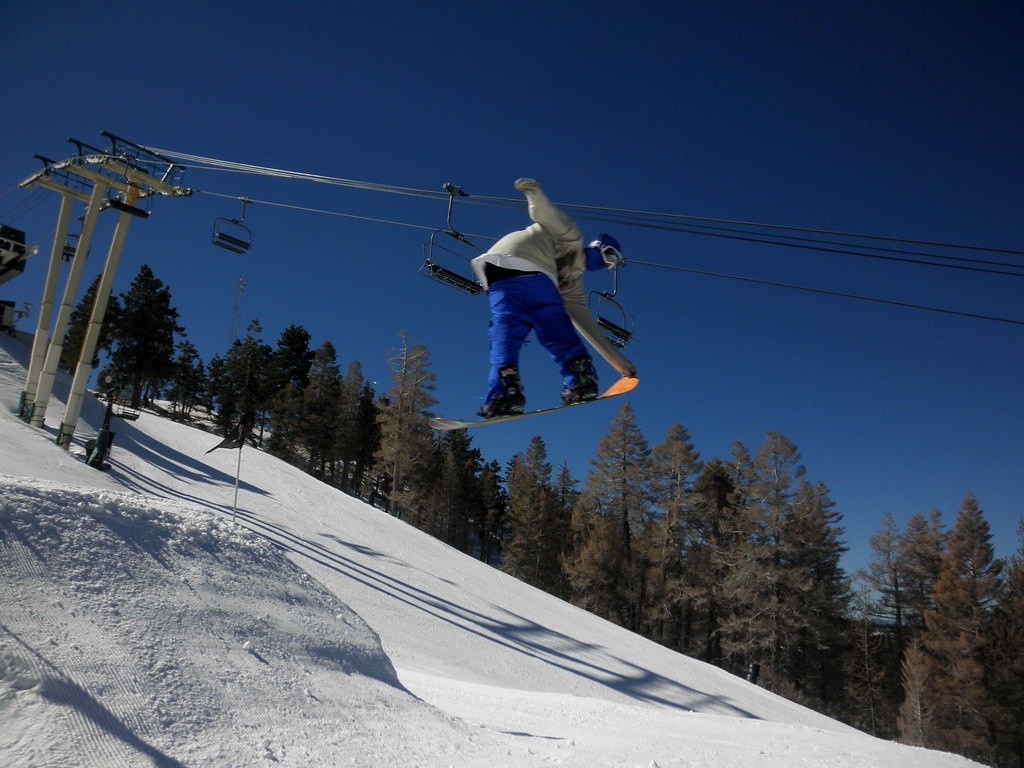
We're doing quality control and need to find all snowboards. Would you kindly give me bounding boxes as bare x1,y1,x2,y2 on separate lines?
427,374,641,432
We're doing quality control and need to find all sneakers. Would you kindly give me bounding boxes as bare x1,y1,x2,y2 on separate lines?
482,364,525,416
560,355,599,402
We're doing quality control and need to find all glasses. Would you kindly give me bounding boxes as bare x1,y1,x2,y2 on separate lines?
602,246,622,270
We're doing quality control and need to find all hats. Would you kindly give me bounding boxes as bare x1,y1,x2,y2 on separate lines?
584,233,620,272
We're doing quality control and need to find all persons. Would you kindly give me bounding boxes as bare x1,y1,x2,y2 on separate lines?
469,177,624,418
